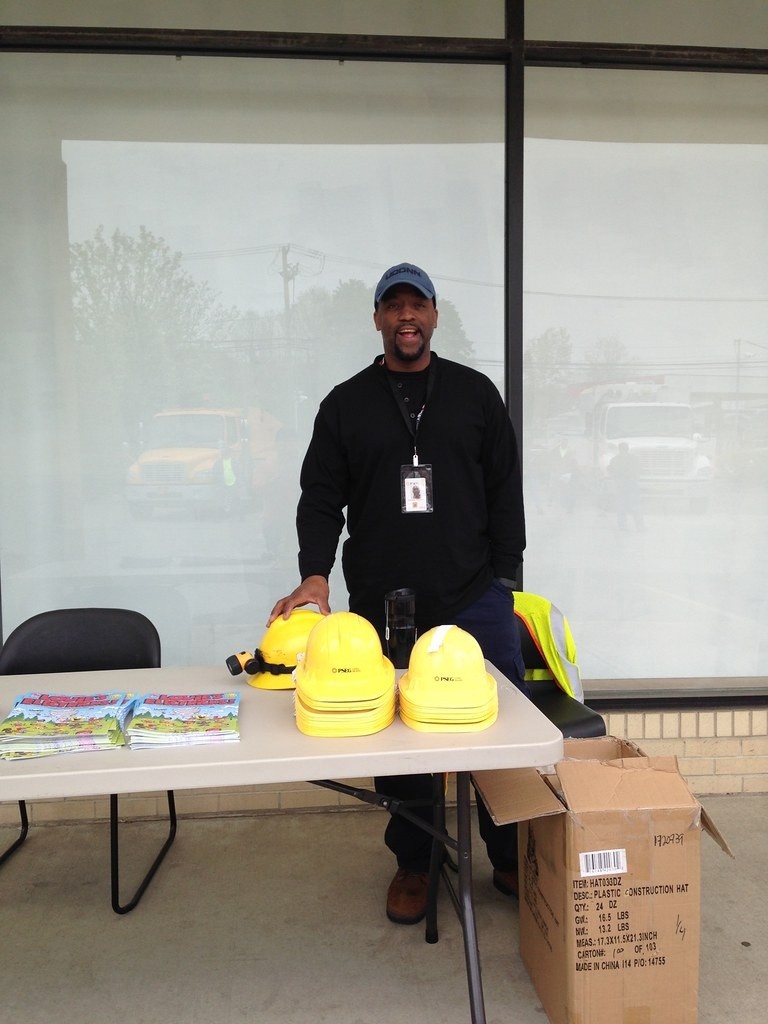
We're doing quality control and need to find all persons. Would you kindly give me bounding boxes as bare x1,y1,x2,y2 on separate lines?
265,263,524,928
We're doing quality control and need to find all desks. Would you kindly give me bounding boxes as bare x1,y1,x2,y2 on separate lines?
1,659,567,1024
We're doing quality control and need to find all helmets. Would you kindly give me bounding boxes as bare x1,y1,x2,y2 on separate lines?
246,609,326,690
295,612,498,737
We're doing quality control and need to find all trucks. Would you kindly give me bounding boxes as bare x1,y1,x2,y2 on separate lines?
581,384,717,519
124,404,291,523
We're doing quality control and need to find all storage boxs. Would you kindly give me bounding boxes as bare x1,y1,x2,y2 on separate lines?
452,737,736,1023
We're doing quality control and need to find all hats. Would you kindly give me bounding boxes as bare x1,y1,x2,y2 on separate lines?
374,263,437,312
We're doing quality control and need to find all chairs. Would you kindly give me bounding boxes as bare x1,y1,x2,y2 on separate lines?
505,590,611,741
0,608,177,913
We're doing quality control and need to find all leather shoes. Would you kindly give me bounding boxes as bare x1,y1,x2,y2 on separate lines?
492,868,520,900
386,866,430,925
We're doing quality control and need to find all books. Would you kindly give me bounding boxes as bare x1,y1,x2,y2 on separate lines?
0,689,240,761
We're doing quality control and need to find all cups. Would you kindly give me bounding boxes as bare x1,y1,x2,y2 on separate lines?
383,588,416,670
